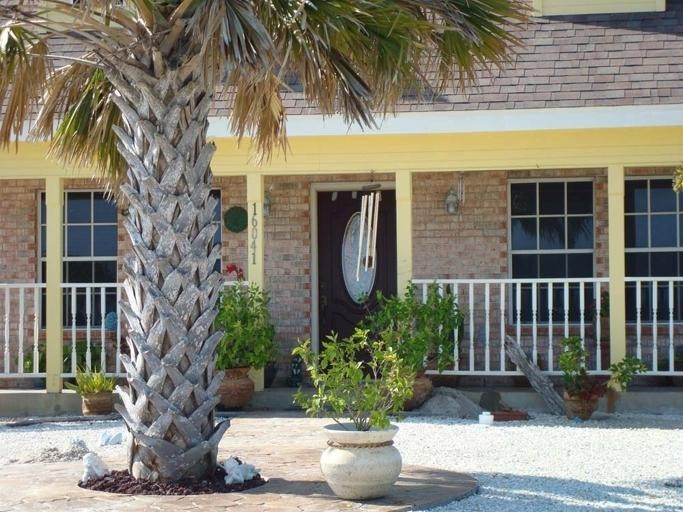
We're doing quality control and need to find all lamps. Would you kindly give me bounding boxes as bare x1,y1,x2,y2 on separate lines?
444,171,465,216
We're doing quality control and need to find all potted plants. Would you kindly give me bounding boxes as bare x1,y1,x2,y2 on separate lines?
63,364,119,416
558,292,646,421
211,282,275,409
290,279,465,501
